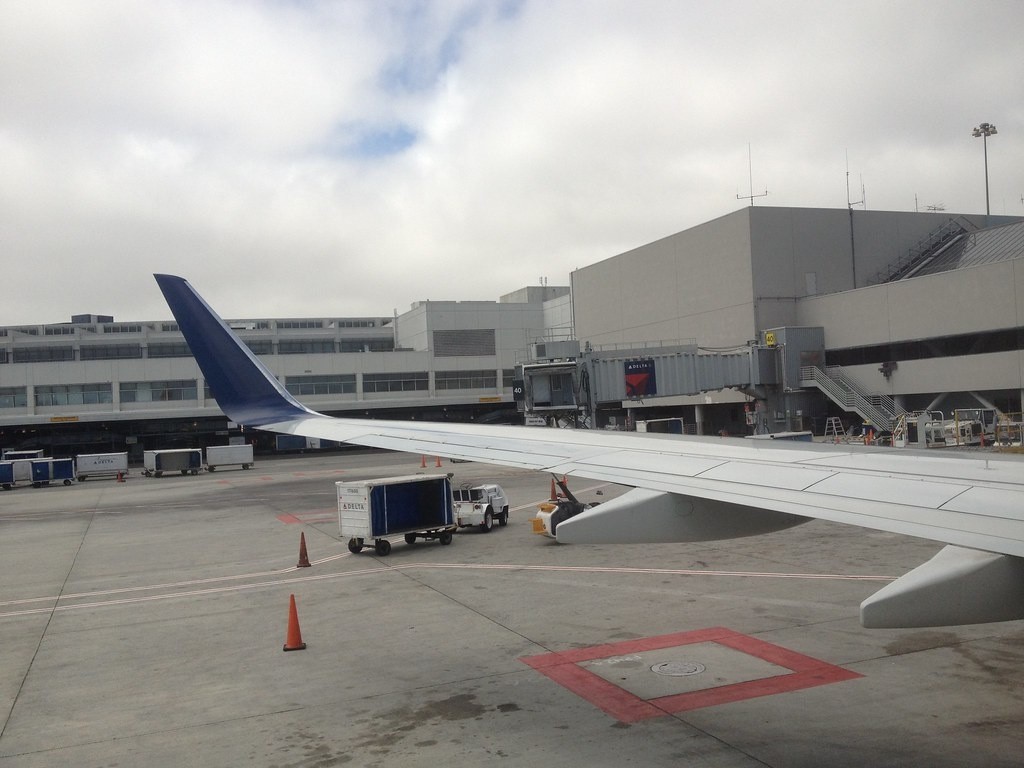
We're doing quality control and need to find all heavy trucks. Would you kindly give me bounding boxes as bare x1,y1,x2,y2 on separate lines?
334,468,510,555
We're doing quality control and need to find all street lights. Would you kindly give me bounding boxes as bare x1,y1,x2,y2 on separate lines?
971,122,997,216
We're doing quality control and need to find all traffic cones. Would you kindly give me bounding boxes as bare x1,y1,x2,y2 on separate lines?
283,594,306,651
549,478,561,501
560,475,568,498
435,456,442,467
295,531,311,568
117,471,124,482
419,454,427,468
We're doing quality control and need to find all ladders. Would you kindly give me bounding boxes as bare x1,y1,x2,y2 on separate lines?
824,417,847,442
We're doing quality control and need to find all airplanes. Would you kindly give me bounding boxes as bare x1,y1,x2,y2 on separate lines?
153,271,1024,630
